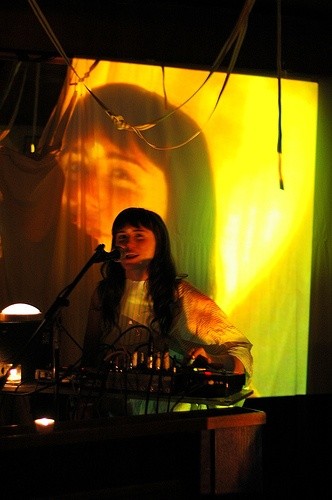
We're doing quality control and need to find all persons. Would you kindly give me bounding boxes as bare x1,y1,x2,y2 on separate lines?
81,206,253,416
49,83,216,416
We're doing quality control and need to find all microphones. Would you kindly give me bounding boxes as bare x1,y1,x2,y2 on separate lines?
93,248,125,263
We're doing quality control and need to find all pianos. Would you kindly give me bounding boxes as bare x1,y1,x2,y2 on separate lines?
1,360,246,406
